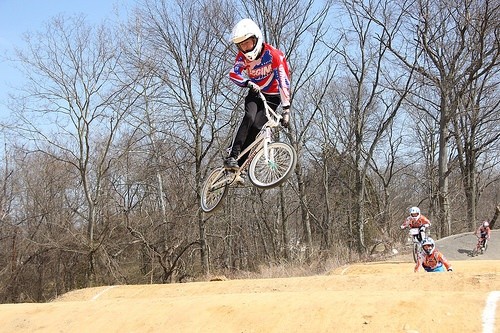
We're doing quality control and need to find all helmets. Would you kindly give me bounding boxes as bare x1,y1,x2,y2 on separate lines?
420,237,435,256
229,18,264,62
483,222,489,228
410,207,420,220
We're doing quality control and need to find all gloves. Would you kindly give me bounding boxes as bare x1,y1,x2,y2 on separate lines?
281,109,290,128
247,80,261,94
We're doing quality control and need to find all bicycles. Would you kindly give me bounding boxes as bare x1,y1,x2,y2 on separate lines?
401,226,426,263
200,85,298,215
472,234,487,257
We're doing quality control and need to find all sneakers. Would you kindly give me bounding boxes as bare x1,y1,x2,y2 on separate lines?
223,156,239,170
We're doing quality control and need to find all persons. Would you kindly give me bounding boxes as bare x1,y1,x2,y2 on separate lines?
475,222,491,254
400,206,432,243
414,237,453,273
223,18,292,172
